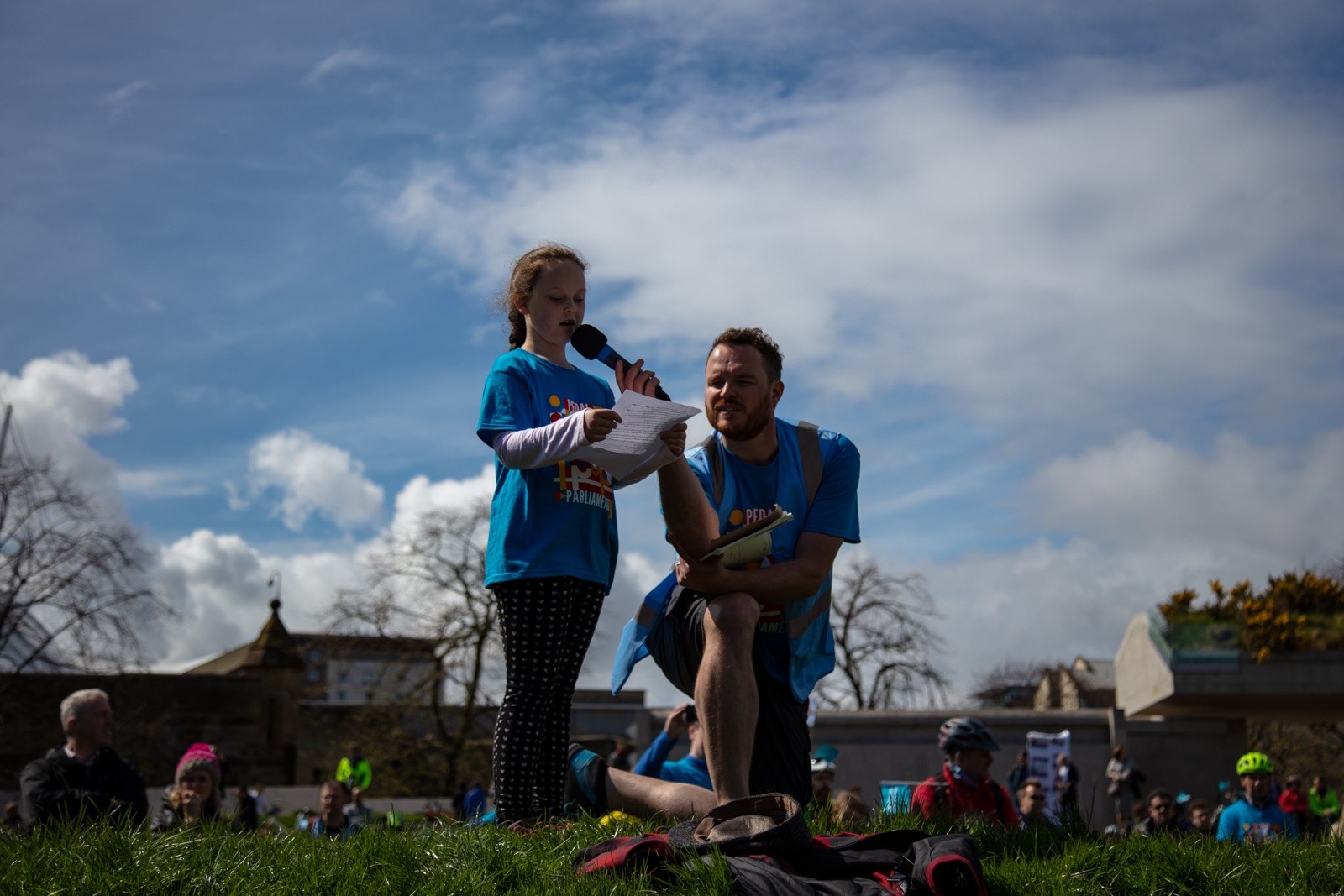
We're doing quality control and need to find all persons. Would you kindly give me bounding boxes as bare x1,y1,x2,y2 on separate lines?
1103,744,1344,849
567,328,861,825
16,688,150,834
149,743,224,835
632,705,715,792
910,717,1021,841
231,781,369,843
476,245,687,829
1008,750,1080,844
809,760,869,826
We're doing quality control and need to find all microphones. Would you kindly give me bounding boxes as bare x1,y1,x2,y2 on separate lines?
570,324,672,402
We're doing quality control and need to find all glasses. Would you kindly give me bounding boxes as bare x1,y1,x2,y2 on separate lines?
1021,794,1046,801
1150,804,1170,810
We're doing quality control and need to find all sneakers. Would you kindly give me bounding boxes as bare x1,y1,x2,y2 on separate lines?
471,742,608,826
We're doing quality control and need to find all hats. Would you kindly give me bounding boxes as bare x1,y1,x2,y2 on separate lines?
666,792,808,854
175,743,221,806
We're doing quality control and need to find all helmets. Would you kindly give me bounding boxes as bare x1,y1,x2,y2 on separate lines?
937,716,1002,754
811,758,837,773
1236,752,1274,776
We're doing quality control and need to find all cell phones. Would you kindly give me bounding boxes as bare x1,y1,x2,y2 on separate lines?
686,706,697,722
